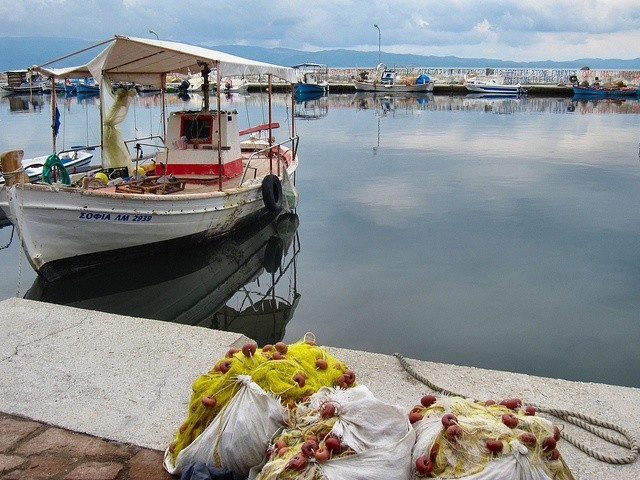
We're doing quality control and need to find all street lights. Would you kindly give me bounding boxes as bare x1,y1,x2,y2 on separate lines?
374,24,380,64
149,31,158,40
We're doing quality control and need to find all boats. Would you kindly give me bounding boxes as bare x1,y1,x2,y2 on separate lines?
65,84,77,91
572,94,640,102
291,62,329,93
350,91,436,109
220,93,250,104
464,93,527,99
464,84,528,93
2,92,45,113
166,82,217,92
353,79,436,91
0,31,300,271
76,81,101,92
77,92,99,103
0,149,93,184
22,207,300,348
65,92,77,100
291,91,329,120
219,79,252,92
1,70,42,92
573,85,640,95
39,80,65,91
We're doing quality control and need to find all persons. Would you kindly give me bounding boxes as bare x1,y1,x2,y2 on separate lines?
224,82,230,93
359,71,368,81
